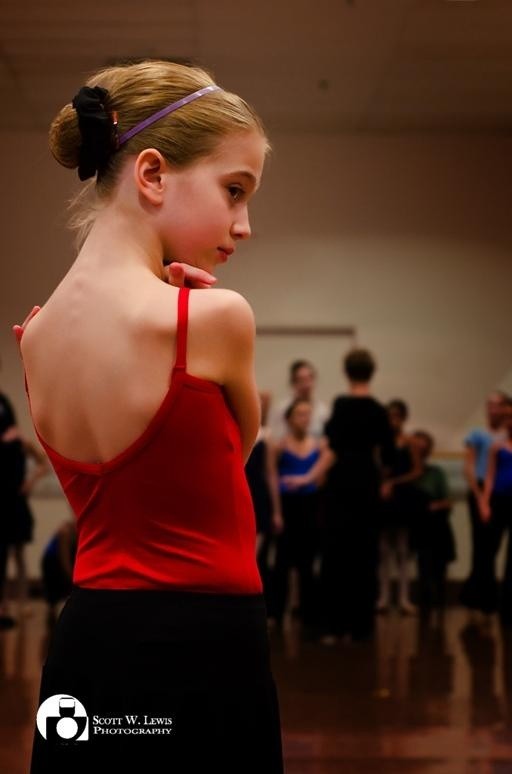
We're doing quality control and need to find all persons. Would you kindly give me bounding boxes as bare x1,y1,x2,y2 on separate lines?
2,632,511,772
12,59,285,771
1,347,511,631
